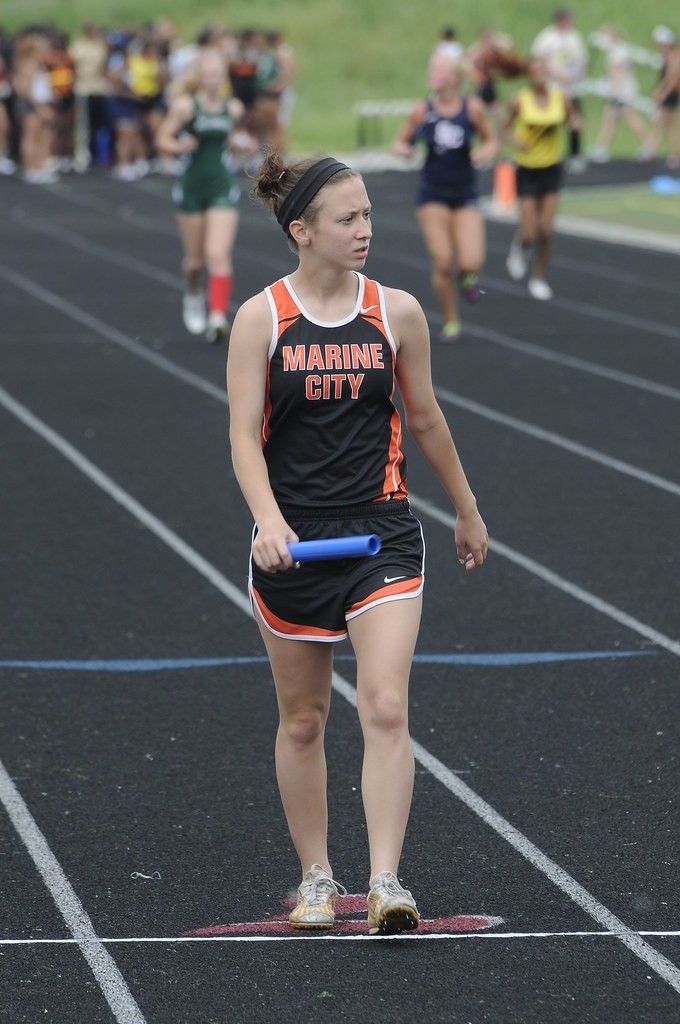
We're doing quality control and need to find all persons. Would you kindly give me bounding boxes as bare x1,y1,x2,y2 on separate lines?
392,41,499,341
433,3,680,193
226,158,491,933
502,55,582,300
0,10,301,185
157,50,253,342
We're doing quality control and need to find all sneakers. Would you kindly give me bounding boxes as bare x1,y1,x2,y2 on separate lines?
289,864,347,930
367,871,421,935
182,292,208,335
438,320,463,343
459,270,486,303
206,315,228,344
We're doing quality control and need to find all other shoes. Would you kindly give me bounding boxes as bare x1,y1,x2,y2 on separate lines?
508,236,529,281
528,278,553,300
